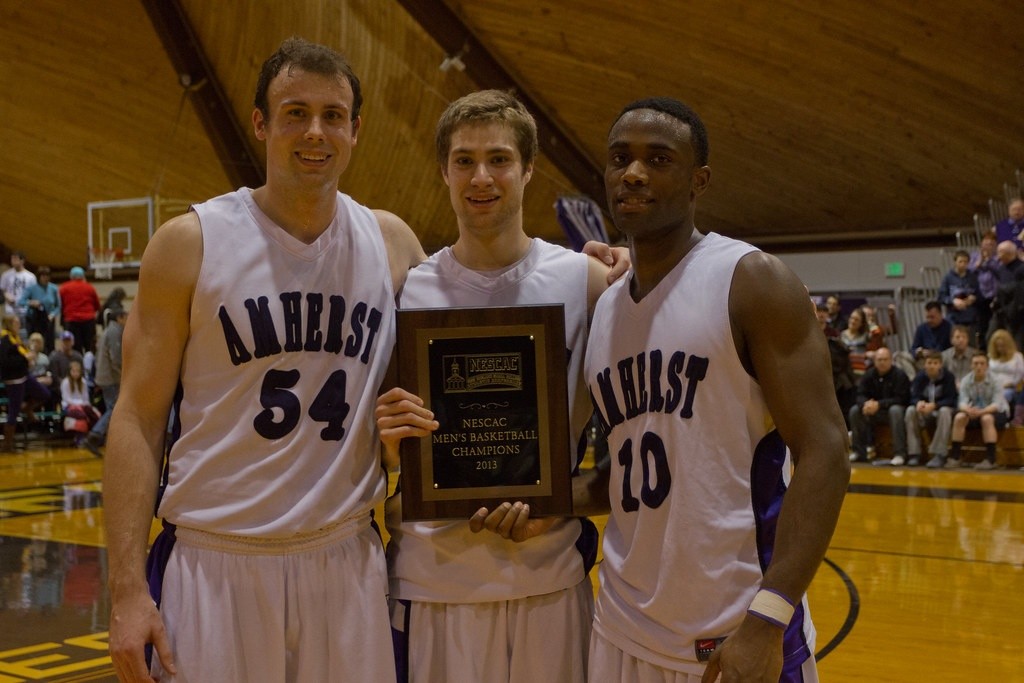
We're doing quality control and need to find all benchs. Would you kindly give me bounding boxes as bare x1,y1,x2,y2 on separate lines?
870,419,1024,468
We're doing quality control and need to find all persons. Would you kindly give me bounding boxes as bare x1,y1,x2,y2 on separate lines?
101,41,633,683
0,252,128,458
375,88,613,682
468,95,851,683
909,199,1023,359
815,292,1024,470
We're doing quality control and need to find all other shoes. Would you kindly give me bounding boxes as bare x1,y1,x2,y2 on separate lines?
850,452,867,462
943,459,961,468
926,456,943,468
907,457,922,465
84,433,105,458
974,459,998,469
890,455,906,465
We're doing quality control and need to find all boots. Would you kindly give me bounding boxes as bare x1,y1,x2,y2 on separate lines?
2,424,23,454
20,400,43,423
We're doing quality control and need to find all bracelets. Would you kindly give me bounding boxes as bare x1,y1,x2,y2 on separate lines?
749,585,795,628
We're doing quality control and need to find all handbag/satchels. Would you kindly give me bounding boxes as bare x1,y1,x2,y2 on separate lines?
25,303,51,340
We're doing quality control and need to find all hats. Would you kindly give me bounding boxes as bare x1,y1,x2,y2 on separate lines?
60,329,76,346
